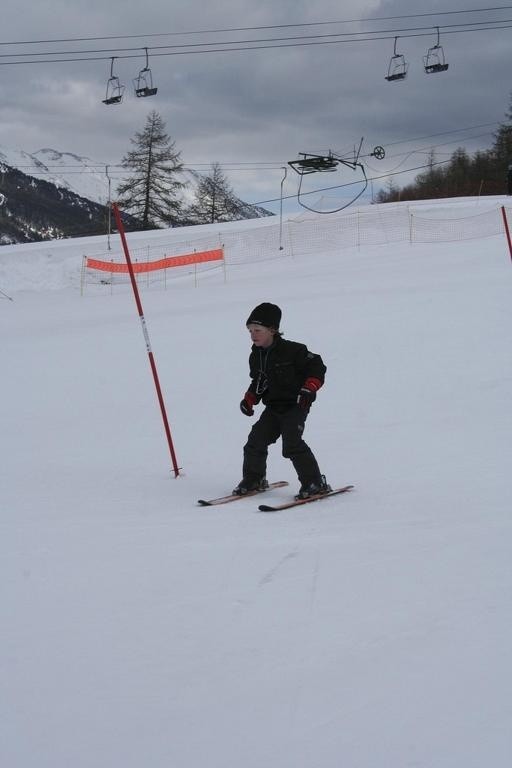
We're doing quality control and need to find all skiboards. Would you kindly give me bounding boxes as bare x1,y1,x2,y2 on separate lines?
198,481,354,511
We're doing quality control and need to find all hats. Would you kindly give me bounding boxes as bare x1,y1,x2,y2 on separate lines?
246,302,281,330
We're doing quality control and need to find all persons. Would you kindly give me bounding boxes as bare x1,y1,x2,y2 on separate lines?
232,302,331,500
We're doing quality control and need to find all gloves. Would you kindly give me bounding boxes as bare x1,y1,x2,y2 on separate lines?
297,376,321,411
240,392,257,416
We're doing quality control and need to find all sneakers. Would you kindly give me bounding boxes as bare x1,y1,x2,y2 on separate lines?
232,476,269,496
295,475,332,502
